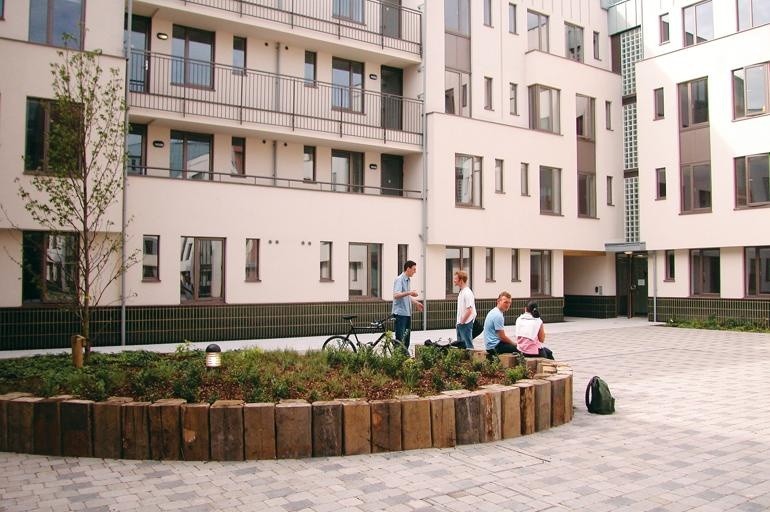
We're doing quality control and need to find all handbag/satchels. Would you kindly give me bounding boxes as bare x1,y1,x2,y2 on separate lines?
471,320,483,339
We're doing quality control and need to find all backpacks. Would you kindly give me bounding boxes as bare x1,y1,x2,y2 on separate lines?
586,376,615,415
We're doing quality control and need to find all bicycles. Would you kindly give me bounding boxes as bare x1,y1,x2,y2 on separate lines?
322,313,410,359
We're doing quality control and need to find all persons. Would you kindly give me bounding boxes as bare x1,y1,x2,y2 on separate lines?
482,291,517,352
389,259,425,350
452,270,478,351
514,299,556,360
